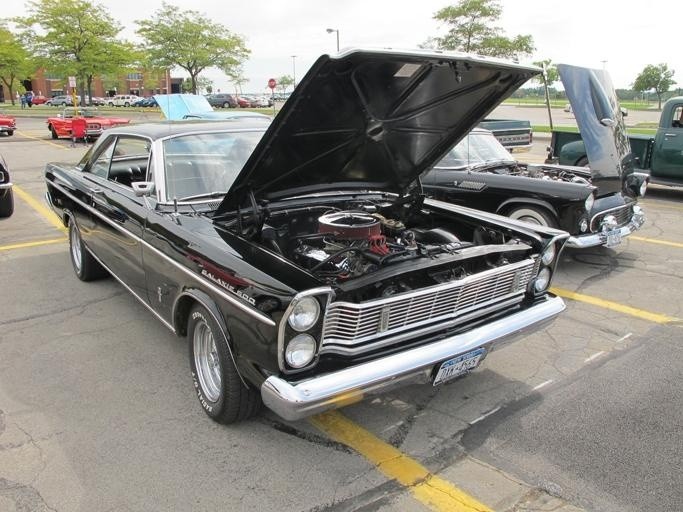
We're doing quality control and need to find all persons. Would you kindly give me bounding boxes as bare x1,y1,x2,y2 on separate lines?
21,93,27,109
27,93,33,108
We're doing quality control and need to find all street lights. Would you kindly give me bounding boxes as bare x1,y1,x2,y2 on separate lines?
292,56,297,90
327,28,340,52
600,60,608,70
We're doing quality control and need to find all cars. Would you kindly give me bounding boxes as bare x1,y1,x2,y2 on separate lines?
44,44,568,425
